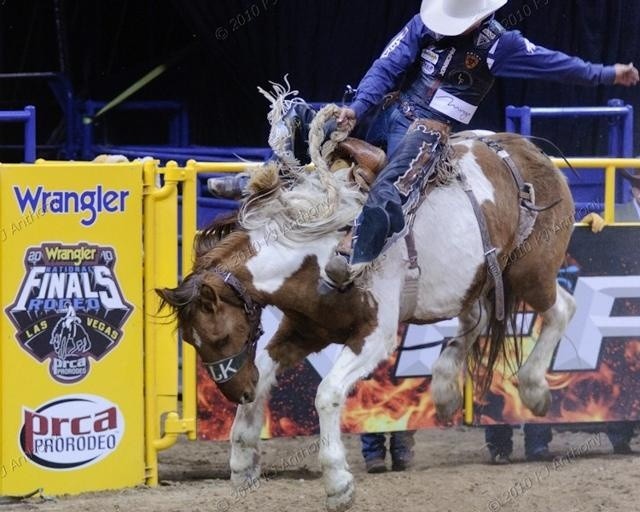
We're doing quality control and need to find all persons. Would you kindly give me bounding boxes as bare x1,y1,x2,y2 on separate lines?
202,1,640,295
599,166,640,225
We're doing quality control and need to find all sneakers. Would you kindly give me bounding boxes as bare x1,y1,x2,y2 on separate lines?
491,450,562,465
365,457,411,474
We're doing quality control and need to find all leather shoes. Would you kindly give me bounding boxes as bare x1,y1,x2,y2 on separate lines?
209,176,250,201
319,254,355,295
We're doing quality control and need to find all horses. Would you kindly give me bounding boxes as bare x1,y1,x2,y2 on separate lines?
155,129,577,512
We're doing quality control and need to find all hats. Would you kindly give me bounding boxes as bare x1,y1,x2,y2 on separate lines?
420,0,508,37
